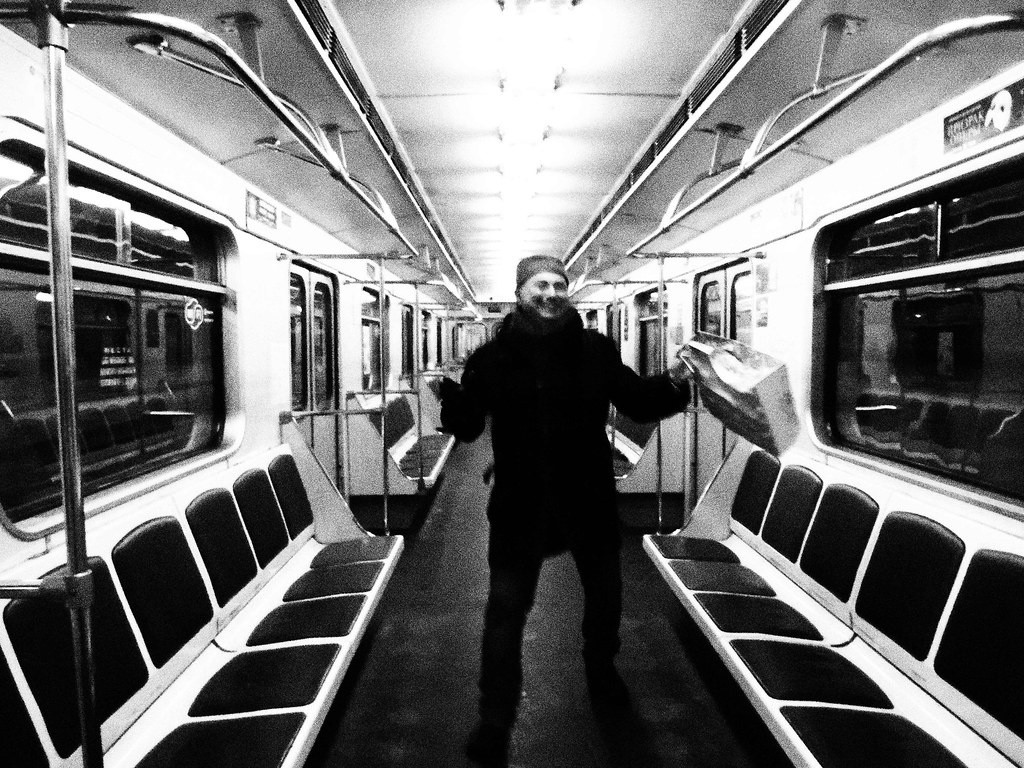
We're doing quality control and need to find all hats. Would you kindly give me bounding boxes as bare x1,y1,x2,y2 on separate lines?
516,255,569,289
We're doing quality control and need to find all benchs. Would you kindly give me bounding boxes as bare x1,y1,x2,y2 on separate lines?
856,387,1024,471
606,404,655,480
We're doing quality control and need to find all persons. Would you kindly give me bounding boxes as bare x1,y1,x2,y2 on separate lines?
425,255,710,768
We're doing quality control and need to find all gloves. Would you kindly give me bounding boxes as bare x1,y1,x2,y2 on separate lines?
438,371,485,442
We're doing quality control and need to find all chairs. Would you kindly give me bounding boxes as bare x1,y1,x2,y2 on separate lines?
4,443,406,768
364,371,455,489
638,444,1023,768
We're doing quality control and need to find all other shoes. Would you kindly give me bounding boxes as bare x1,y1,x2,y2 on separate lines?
584,661,625,711
466,709,511,766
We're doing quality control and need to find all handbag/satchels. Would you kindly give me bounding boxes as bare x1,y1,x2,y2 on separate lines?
676,330,800,455
